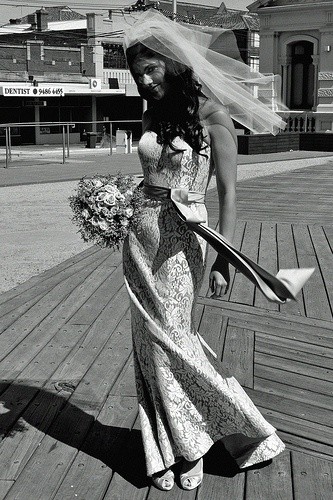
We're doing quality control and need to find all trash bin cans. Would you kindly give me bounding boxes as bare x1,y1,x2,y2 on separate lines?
86,131,98,149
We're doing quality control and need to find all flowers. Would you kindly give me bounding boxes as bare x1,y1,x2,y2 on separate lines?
67,169,148,252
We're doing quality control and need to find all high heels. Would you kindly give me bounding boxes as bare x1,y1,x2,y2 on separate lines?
180,455,204,490
151,468,175,490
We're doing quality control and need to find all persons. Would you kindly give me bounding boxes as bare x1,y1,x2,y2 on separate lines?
112,25,239,491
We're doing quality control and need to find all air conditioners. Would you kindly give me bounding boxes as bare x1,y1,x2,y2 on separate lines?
90,78,101,89
